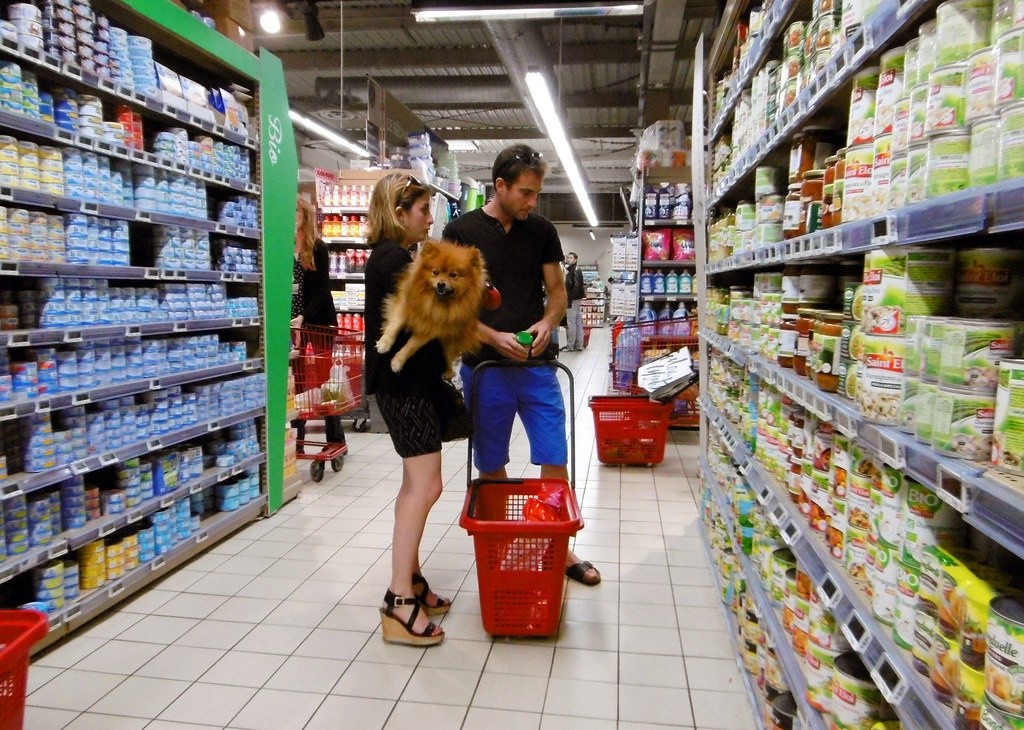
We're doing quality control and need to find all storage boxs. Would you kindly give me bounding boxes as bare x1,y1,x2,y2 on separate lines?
140,443,203,496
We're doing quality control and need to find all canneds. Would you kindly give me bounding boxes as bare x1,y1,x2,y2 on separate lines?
698,0,1024,730
0,0,260,623
514,331,533,353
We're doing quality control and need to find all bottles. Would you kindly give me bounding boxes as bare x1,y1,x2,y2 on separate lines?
640,269,700,337
516,331,533,354
480,281,501,310
321,183,374,333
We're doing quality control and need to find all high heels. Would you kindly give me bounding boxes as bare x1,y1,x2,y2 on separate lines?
380,589,444,646
412,574,450,615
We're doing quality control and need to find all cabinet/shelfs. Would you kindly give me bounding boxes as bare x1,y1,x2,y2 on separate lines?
695,0,1024,730
632,166,702,432
0,32,264,661
313,205,378,420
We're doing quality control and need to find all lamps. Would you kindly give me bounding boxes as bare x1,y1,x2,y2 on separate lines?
302,0,325,41
589,230,596,241
572,224,624,229
289,102,373,158
523,65,601,229
409,0,645,26
610,235,613,244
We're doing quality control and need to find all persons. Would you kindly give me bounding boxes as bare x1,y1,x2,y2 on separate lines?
364,173,456,647
441,142,601,588
558,252,587,353
290,196,347,458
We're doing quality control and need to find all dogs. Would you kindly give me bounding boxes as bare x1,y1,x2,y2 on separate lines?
377,240,485,378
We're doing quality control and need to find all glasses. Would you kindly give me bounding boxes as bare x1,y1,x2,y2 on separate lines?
396,173,422,207
496,151,543,177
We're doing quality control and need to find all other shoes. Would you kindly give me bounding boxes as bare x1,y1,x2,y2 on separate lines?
575,348,581,351
561,346,571,352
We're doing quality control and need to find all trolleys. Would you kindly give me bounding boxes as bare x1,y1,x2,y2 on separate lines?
607,315,698,428
288,321,367,482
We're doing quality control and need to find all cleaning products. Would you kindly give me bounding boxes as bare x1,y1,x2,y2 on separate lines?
638,181,699,337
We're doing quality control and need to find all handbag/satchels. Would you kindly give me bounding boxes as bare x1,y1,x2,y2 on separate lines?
637,346,695,400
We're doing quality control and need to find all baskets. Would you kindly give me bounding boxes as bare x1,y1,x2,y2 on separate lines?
461,358,583,637
1,608,49,730
589,393,675,466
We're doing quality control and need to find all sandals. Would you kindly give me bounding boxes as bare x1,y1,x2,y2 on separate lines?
566,561,601,586
